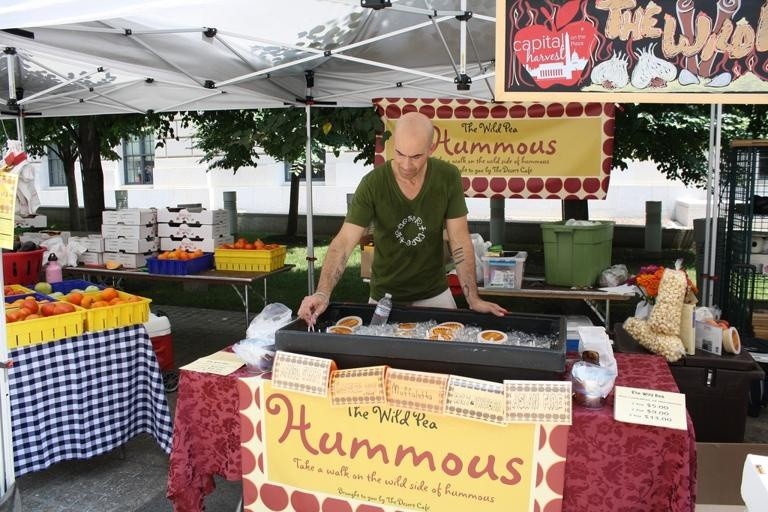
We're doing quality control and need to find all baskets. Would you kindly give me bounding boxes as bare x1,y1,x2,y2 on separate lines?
2,246,48,285
4,278,152,350
146,250,213,274
213,244,287,273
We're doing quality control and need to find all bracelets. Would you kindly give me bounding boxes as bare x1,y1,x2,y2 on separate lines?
310,291,330,302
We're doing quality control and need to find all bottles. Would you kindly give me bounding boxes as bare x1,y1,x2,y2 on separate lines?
575,351,607,409
369,293,392,329
46,253,63,284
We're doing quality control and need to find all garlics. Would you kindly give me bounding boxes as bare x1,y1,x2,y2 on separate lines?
679,70,699,85
706,73,731,88
631,43,678,90
591,50,628,88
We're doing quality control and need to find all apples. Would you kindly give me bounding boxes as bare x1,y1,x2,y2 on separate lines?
220,237,279,250
4,282,142,324
514,5,597,89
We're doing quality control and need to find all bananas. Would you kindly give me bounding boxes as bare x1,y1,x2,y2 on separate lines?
106,262,123,269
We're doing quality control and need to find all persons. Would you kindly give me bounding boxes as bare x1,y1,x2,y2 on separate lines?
297,112,509,327
